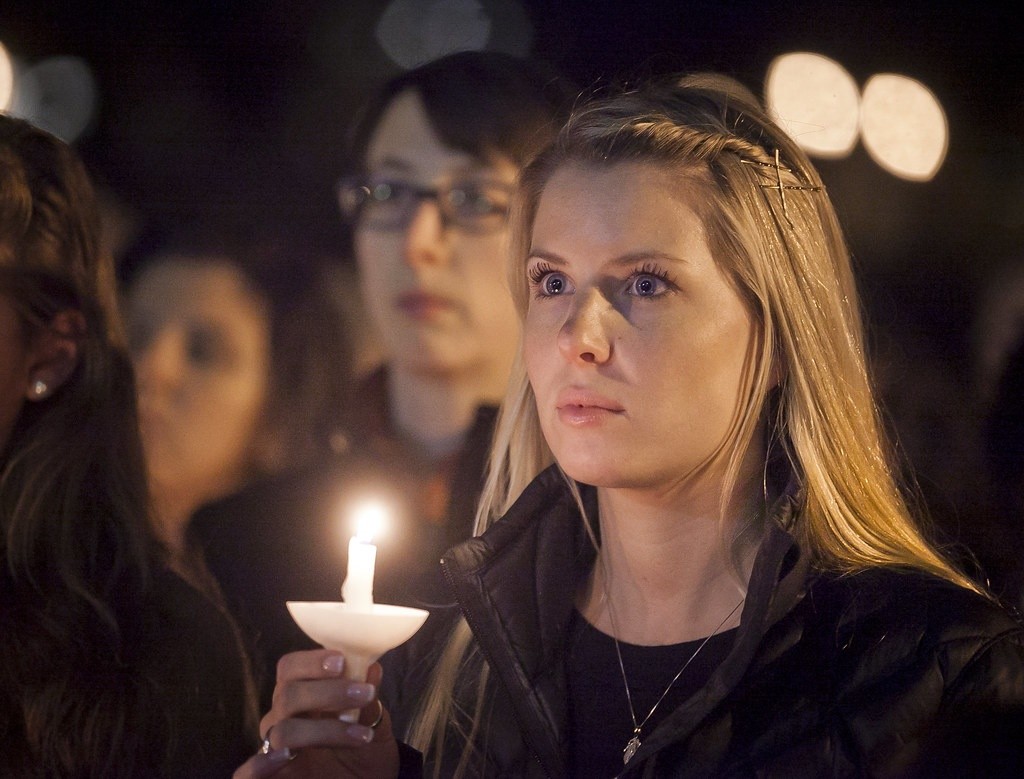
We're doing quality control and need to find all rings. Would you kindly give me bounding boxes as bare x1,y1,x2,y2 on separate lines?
370,700,384,728
263,728,297,760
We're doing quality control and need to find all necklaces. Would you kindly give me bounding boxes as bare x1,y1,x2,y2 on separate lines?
599,559,744,765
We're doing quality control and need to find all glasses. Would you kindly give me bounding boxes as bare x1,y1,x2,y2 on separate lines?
337,172,517,236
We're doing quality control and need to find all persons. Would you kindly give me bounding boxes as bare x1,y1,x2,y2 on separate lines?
195,49,569,747
123,248,294,715
231,78,1024,779
0,112,263,779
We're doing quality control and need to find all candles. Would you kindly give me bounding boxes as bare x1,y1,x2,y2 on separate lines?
338,495,378,602
